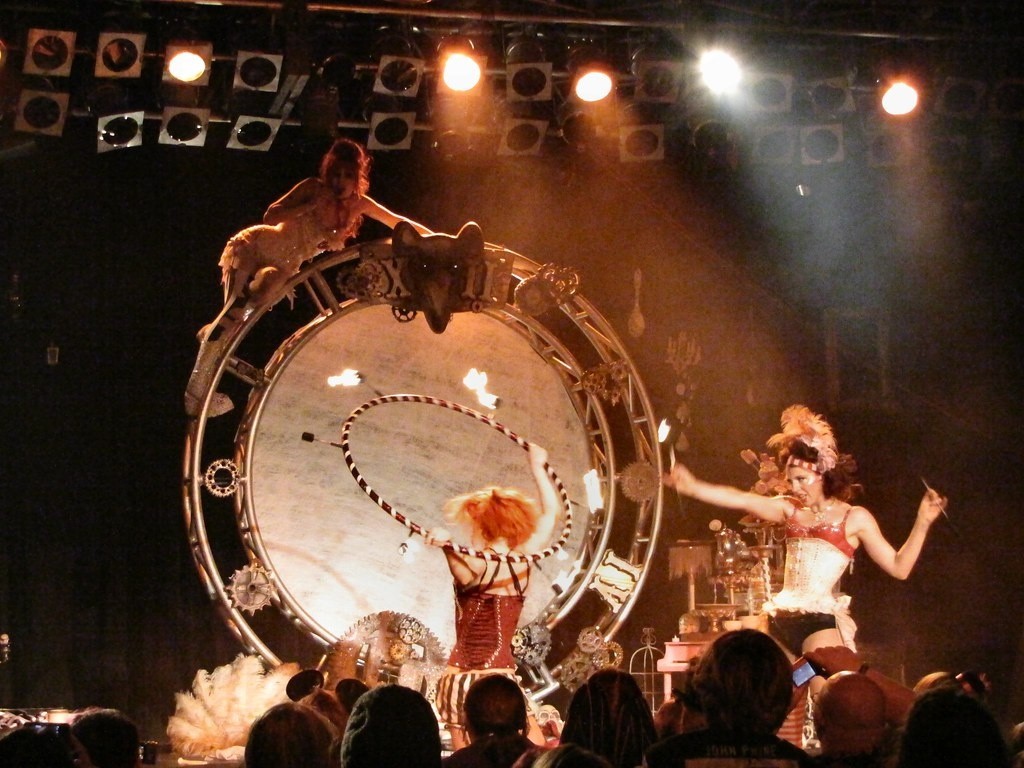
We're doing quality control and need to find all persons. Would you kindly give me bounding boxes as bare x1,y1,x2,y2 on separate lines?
659,405,948,750
424,441,559,753
513,668,660,768
245,689,350,768
440,674,545,768
185,139,436,417
811,664,1024,768
657,629,815,768
340,684,441,768
0,709,139,768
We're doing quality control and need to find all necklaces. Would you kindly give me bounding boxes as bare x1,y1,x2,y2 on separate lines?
804,498,837,523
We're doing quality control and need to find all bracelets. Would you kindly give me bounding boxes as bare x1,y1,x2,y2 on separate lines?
859,663,869,674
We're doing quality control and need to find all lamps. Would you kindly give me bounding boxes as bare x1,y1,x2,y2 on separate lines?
0,0,1023,179
